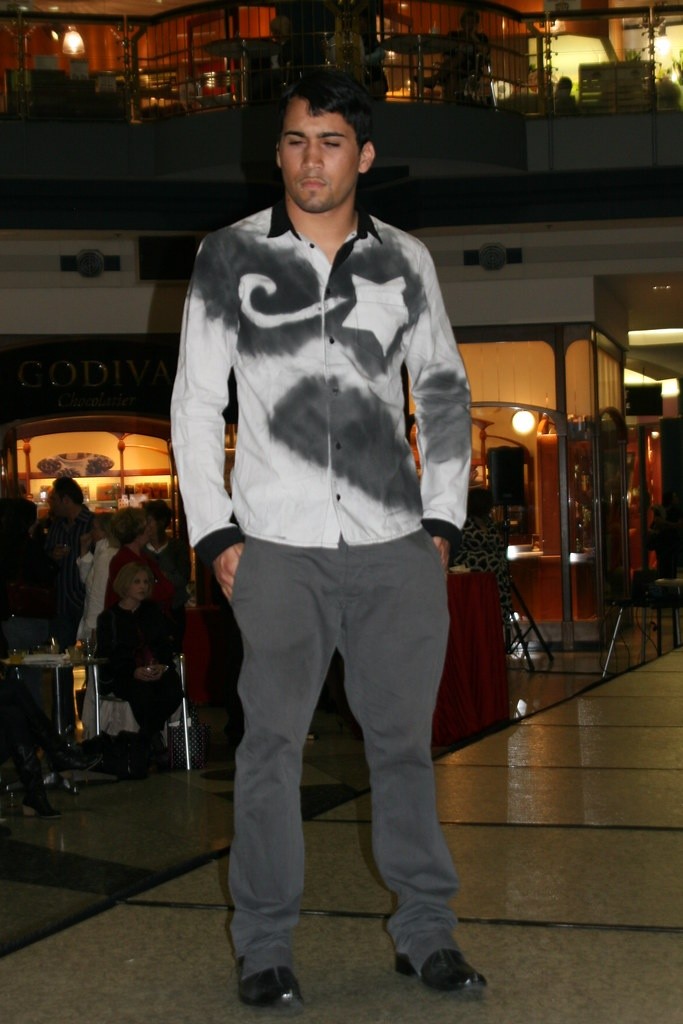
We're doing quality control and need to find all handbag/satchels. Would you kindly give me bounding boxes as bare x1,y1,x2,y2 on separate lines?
167,700,209,768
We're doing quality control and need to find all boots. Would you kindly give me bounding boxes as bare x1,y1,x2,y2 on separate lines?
34,710,106,779
11,745,62,819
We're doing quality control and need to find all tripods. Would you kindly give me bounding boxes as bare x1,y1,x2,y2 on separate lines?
500,506,554,674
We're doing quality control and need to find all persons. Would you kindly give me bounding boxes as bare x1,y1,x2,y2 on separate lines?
210,574,364,752
0,478,190,819
170,73,486,1009
554,78,576,114
412,10,490,98
452,488,512,627
653,491,683,608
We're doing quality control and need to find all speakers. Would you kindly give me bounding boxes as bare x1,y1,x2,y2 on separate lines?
488,448,525,507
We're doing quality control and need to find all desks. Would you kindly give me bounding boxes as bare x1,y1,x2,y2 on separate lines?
204,37,279,103
379,33,448,102
504,553,595,652
430,570,509,747
0,653,110,795
181,604,239,707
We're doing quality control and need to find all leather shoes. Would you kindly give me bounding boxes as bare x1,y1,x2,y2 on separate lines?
239,961,305,1016
393,948,486,992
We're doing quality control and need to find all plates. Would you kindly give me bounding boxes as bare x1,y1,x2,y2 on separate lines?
3,653,69,663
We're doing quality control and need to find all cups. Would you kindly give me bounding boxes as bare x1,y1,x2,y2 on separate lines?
68,638,84,661
146,659,163,680
32,644,48,654
86,630,98,659
7,648,29,665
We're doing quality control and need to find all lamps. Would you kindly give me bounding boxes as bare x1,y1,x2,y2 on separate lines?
51,25,65,41
62,25,85,54
